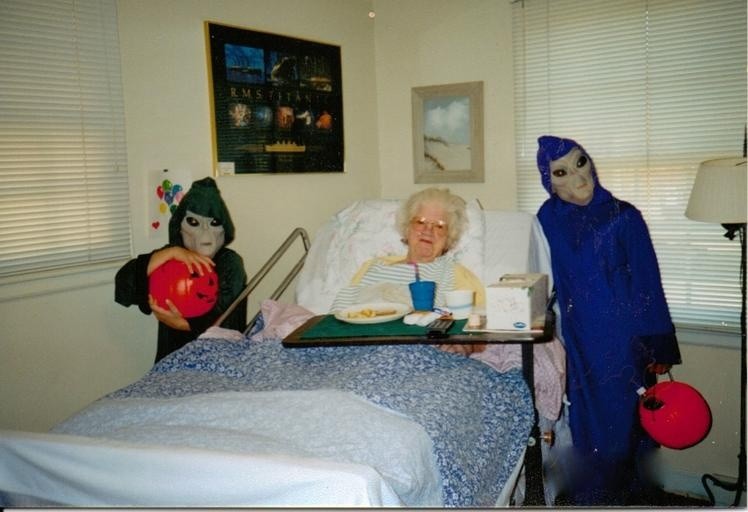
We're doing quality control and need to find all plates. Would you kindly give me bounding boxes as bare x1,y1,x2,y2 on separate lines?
334,302,409,324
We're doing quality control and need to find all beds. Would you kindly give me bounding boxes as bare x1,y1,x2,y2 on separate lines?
1,194,552,511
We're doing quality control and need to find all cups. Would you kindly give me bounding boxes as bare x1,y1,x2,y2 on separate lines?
410,281,437,312
446,289,474,318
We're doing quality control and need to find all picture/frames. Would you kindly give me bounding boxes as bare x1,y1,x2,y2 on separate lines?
410,81,487,185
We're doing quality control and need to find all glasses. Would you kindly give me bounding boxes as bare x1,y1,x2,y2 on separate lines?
411,216,449,237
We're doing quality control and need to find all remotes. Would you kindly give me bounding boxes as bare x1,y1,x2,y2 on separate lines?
426,318,455,337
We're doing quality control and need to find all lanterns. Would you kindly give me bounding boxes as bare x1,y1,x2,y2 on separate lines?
638,360,713,448
147,254,221,320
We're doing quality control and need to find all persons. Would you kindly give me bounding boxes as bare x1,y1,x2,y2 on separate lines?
326,188,490,359
530,130,683,508
112,175,249,369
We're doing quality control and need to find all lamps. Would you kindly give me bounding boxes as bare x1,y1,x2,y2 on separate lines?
676,157,748,507
